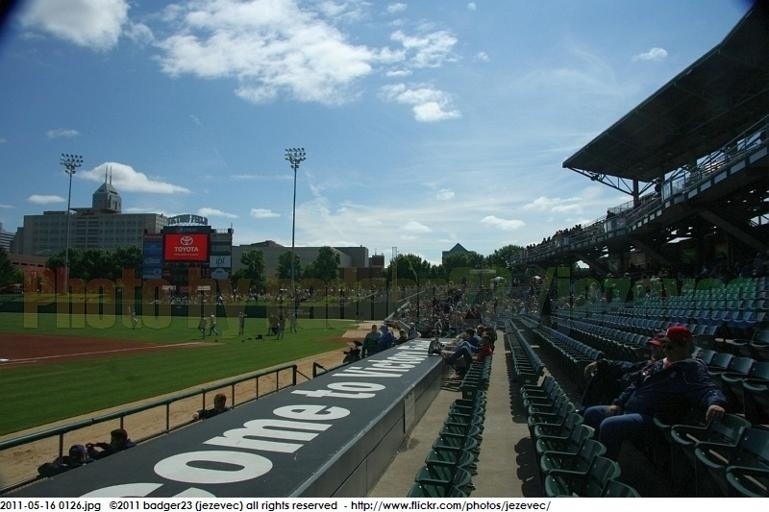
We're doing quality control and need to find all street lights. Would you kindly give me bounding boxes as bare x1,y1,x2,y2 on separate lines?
283,147,307,298
58,151,83,295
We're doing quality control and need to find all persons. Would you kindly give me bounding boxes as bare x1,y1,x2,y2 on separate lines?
85,428,137,462
37,444,92,480
129,312,140,329
150,239,769,464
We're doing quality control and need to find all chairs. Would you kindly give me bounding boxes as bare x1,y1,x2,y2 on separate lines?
404,276,768,497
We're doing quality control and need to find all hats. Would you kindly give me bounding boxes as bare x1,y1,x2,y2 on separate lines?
647,326,690,347
